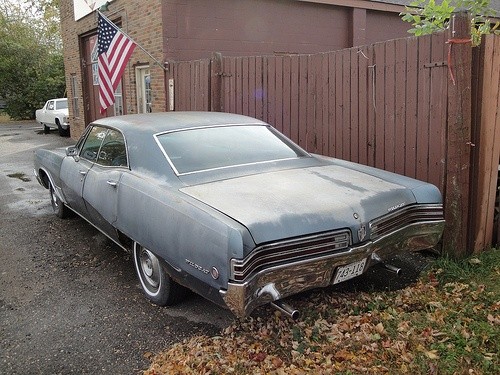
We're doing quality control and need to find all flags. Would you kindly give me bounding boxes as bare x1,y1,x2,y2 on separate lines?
97,12,137,117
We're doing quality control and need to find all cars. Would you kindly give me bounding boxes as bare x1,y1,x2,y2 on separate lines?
35,97,68,137
31,109,449,323
